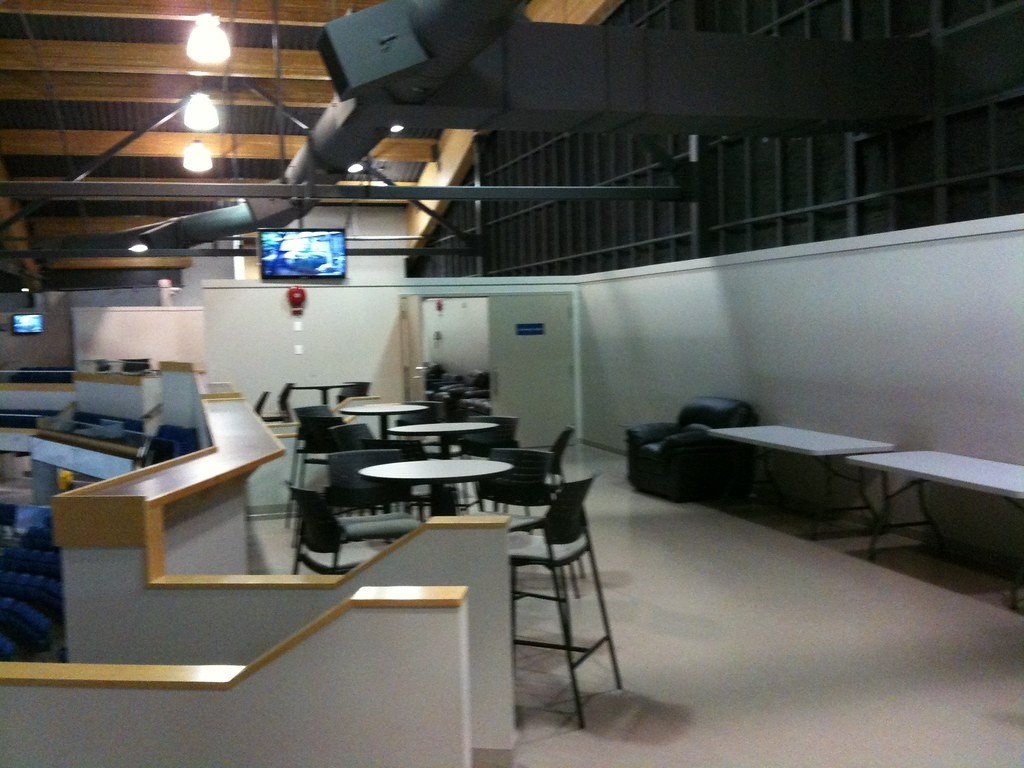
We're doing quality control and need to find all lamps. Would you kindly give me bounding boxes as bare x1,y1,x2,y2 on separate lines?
183,91,218,133
183,139,214,173
184,12,233,67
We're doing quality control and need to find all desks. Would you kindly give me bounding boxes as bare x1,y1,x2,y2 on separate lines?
703,425,896,538
359,459,515,517
291,383,355,405
387,422,500,460
845,450,1023,559
339,402,428,438
80,382,285,505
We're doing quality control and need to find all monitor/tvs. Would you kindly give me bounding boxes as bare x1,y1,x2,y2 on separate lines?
257,227,347,280
11,314,45,334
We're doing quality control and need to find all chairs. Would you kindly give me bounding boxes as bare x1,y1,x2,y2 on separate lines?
336,381,371,403
256,381,295,424
395,401,442,441
490,424,574,534
506,475,623,731
325,422,373,451
321,449,426,544
450,415,532,518
283,405,346,530
360,438,426,524
285,487,425,575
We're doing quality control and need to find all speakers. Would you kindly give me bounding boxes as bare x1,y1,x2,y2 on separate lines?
317,0,428,102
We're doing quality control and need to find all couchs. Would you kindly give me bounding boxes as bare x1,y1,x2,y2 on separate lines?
623,395,762,504
